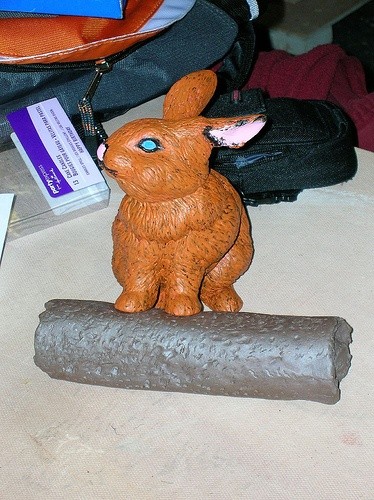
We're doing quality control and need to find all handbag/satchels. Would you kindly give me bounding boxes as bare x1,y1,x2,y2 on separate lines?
200,88,357,205
0,0,256,143
241,44,374,153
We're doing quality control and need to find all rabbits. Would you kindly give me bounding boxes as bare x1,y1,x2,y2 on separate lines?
96,69,266,317
